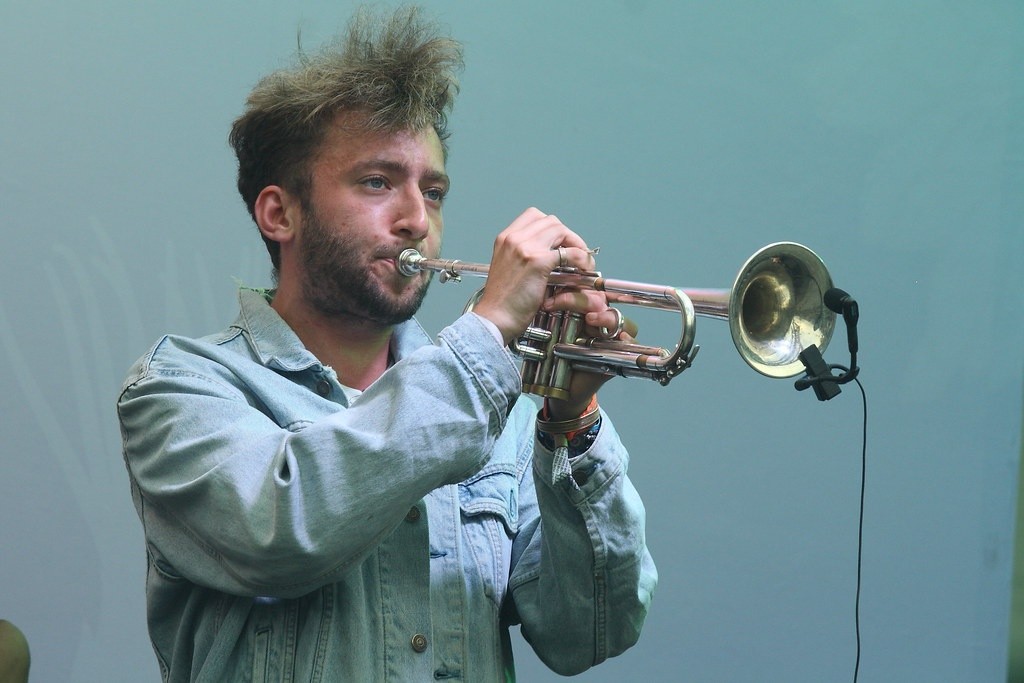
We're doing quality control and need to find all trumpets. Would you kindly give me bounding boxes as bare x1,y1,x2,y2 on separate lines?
393,240,840,402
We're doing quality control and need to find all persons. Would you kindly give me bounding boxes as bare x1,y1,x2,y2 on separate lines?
0,620,31,683
116,0,659,683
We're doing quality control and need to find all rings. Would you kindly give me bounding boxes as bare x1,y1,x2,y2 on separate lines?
558,246,569,267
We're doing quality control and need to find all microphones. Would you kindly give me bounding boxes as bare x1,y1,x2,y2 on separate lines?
824,287,860,353
